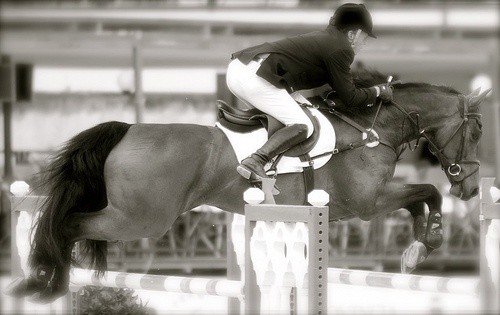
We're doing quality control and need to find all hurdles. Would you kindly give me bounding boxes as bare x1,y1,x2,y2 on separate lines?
219,178,499,315
3,180,330,315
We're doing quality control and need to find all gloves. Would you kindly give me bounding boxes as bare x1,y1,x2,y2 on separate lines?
378,85,394,103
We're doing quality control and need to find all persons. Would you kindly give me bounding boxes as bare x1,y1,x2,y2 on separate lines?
225,2,394,195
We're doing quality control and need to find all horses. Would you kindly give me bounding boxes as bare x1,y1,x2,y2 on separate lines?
3,69,493,305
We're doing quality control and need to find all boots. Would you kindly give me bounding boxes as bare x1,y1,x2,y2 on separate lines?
237,124,308,196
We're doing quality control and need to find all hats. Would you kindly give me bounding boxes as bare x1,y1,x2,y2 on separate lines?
333,3,377,38
